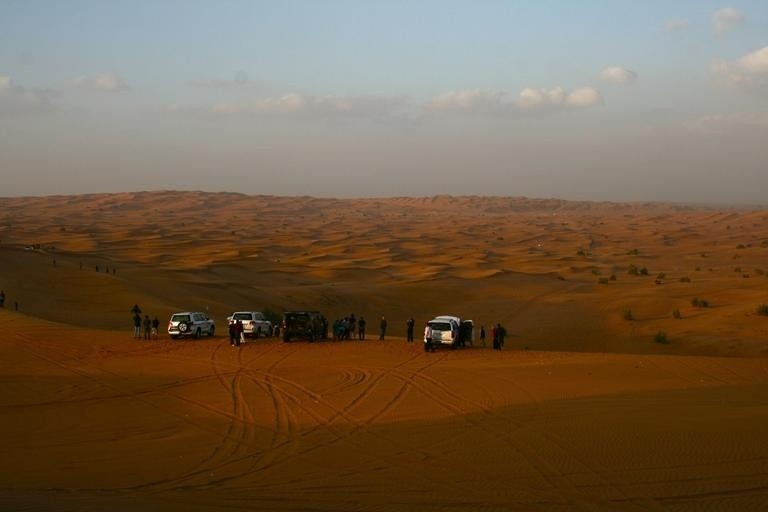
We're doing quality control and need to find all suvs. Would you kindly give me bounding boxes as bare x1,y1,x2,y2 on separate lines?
226,311,274,338
167,311,216,339
423,313,475,349
280,310,329,344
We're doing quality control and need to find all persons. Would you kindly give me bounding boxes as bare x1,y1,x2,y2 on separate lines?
130,303,159,341
479,324,487,347
457,321,472,348
330,312,366,341
378,315,386,339
274,320,280,337
491,323,504,352
406,317,414,343
229,319,243,348
424,321,435,352
